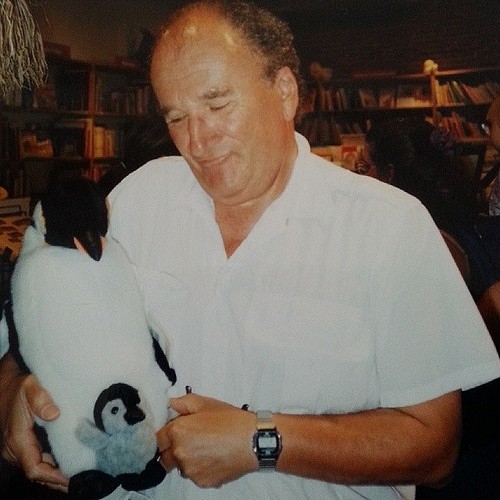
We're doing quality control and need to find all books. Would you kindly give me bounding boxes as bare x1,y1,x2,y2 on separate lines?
9,66,156,193
298,86,353,146
434,78,500,138
357,82,432,108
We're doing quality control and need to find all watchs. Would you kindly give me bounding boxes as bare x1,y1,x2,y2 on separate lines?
251,410,282,468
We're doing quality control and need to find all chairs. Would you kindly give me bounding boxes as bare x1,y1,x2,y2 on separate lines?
438,228,471,282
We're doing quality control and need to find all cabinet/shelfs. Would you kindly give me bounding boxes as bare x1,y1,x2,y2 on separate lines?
0,54,154,197
295,65,500,157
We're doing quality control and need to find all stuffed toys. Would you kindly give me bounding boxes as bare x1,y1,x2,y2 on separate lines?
6,176,178,500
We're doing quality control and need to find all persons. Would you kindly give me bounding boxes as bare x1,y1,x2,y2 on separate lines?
356,95,500,317
0,0,500,500
